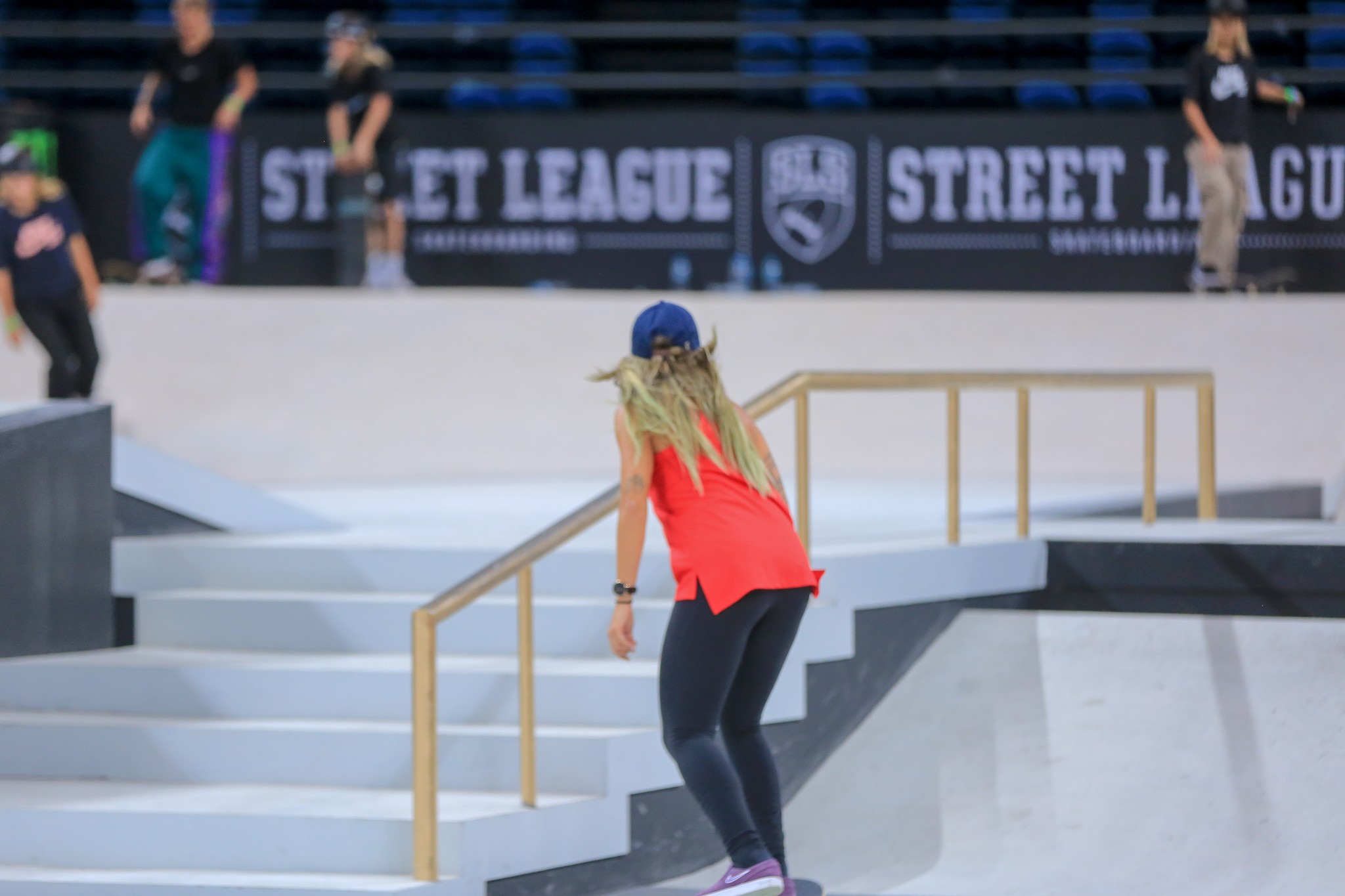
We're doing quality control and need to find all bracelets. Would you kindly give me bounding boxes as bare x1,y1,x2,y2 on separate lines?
1284,86,1298,106
8,315,19,332
222,94,246,113
616,600,632,605
333,139,347,155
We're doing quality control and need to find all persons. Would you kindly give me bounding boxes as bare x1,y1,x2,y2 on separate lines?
590,301,824,896
0,141,101,398
127,2,260,286
1182,12,1304,293
321,10,415,287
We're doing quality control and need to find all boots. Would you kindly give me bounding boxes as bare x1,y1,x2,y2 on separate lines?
367,251,412,288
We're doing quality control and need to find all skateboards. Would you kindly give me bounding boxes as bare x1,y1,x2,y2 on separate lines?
1185,264,1304,295
133,265,184,285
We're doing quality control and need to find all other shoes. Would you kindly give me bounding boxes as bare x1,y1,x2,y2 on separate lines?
1196,263,1235,292
139,257,180,283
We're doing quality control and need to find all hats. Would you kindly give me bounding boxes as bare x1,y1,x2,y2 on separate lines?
631,300,697,360
327,13,370,45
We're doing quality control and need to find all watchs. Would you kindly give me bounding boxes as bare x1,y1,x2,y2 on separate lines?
613,583,636,595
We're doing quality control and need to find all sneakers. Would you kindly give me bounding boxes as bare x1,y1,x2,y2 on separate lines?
695,860,782,896
780,877,796,895
